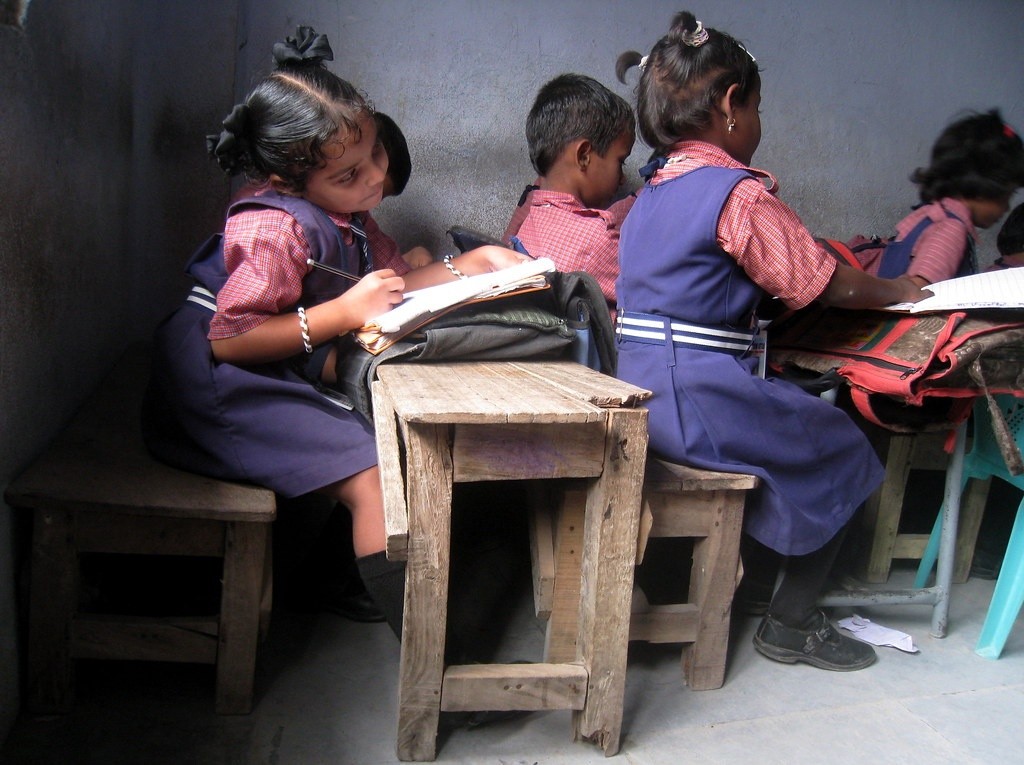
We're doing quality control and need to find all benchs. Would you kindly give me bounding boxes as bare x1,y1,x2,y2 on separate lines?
525,455,762,693
12,339,279,715
853,424,994,585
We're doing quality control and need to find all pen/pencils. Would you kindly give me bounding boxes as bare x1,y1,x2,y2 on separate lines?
305,258,406,295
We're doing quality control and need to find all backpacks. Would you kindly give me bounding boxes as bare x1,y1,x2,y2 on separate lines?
812,233,893,279
772,312,1024,476
334,298,578,431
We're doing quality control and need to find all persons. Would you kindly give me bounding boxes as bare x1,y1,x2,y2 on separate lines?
133,23,642,729
878,104,1024,291
617,12,936,670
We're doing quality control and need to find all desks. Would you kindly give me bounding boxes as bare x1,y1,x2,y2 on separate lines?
366,359,658,761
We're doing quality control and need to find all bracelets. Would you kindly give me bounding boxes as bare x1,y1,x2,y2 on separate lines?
444,252,467,280
298,306,312,356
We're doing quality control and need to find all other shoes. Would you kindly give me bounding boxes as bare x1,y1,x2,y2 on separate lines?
752,614,878,671
439,658,542,732
734,576,776,615
317,577,384,623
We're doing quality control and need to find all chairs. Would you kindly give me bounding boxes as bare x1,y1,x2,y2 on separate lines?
914,392,1024,660
